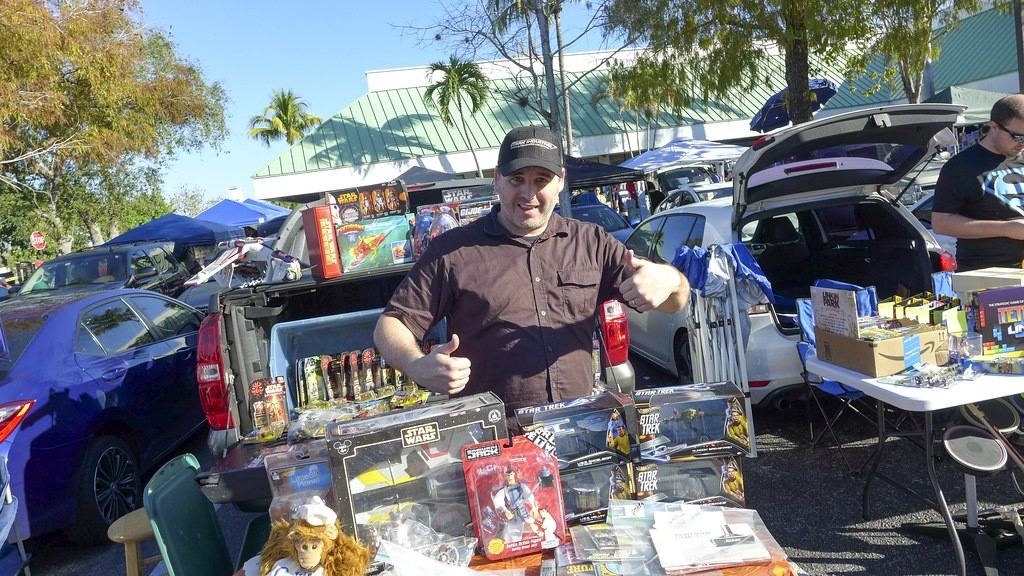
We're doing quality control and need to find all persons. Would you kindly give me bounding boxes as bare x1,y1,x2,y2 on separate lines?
491,468,538,543
718,398,749,502
609,410,630,503
373,124,690,419
637,180,666,218
930,93,1024,275
614,183,631,217
594,186,607,204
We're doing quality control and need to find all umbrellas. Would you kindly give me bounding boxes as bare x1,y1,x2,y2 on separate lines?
750,76,837,134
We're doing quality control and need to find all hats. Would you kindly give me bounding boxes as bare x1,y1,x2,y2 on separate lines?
498,124,564,178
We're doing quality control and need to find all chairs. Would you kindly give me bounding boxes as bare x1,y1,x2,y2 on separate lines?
763,217,797,243
794,277,940,477
71,265,95,284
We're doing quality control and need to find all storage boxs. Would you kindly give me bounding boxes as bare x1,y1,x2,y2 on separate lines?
271,308,445,415
964,351,1024,375
262,390,566,572
235,513,272,572
814,319,945,378
513,379,751,527
299,179,497,279
953,265,1023,306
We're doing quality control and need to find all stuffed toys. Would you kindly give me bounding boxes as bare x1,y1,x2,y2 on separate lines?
230,495,370,576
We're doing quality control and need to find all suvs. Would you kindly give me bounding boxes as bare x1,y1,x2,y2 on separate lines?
14,237,193,297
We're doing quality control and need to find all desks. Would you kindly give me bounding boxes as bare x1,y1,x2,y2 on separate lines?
805,343,1024,576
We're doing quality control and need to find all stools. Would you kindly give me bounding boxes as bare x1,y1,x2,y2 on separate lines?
106,507,163,576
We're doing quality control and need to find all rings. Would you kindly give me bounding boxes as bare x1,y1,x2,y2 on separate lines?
631,299,638,306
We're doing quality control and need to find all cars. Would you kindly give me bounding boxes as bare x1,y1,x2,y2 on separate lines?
906,152,949,193
0,285,10,302
174,269,261,317
619,164,735,215
816,142,894,163
621,104,970,414
844,190,957,261
554,202,635,246
0,290,208,549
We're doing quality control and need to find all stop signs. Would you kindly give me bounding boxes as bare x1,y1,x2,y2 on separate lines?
30,231,46,250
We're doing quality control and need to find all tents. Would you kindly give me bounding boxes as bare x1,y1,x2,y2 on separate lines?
564,154,654,218
387,164,497,212
616,136,751,188
101,198,293,263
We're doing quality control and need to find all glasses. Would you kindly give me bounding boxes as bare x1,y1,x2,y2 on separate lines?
994,120,1024,143
